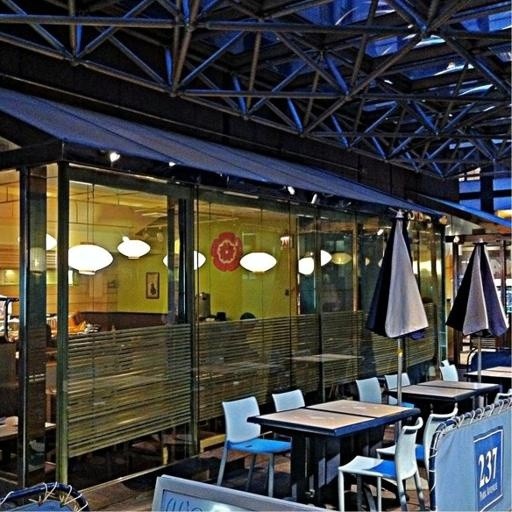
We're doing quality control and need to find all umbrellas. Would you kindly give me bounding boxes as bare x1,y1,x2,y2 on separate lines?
442,238,510,384
364,210,428,439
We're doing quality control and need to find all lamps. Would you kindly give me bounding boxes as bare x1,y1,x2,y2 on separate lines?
67,185,279,275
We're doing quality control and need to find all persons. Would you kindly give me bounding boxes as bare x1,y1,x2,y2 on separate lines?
221,311,265,365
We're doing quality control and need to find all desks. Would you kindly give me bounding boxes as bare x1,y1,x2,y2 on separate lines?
1,341,215,484
